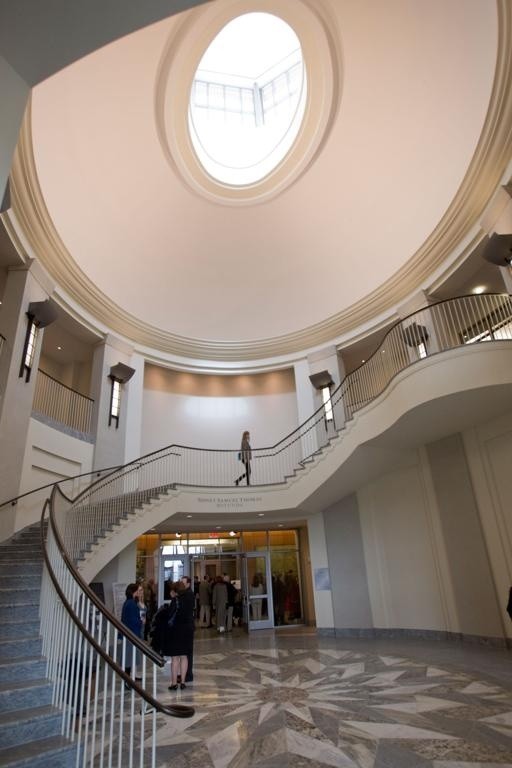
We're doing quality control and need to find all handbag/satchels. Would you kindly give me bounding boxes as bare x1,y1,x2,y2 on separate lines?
239,452,241,460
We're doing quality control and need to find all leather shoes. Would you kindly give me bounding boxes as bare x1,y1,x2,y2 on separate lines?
177,674,193,683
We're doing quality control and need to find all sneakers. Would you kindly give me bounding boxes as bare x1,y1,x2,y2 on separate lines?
124,674,142,690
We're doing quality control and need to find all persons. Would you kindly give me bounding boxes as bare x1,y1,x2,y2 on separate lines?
119,576,195,690
194,573,241,634
249,570,301,624
236,431,252,485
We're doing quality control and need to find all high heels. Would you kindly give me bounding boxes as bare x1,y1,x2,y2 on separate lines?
168,684,186,690
234,479,239,486
